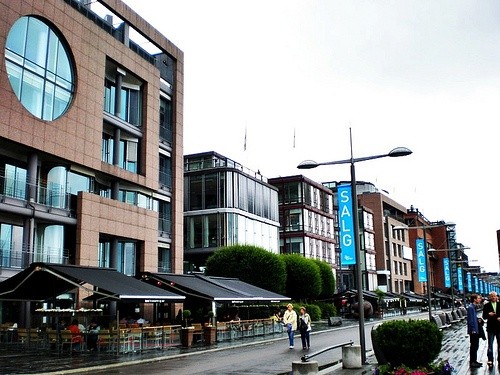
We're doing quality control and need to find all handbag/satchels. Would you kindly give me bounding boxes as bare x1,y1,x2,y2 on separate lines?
284,323,287,327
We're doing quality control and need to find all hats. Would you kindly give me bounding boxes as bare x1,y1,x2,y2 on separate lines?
286,303,294,308
299,306,306,311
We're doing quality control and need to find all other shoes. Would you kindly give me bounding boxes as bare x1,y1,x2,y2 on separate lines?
289,345,294,349
303,347,307,350
487,361,493,367
469,361,483,368
307,347,310,350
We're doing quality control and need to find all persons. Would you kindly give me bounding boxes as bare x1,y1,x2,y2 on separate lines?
223,314,240,325
467,294,486,368
270,313,281,332
299,306,312,349
284,303,297,349
68,319,100,352
482,290,500,365
56,320,66,345
276,312,282,320
401,297,407,315
120,315,145,325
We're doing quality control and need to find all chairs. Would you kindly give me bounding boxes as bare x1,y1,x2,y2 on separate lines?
0,324,184,354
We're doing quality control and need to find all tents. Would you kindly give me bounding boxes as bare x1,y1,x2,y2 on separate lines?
0,262,186,355
82,272,292,340
352,289,448,306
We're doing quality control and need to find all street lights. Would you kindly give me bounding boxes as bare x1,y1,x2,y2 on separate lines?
427,247,500,313
393,220,456,325
296,127,413,365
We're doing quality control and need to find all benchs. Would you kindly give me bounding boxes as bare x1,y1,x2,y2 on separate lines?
431,307,467,332
